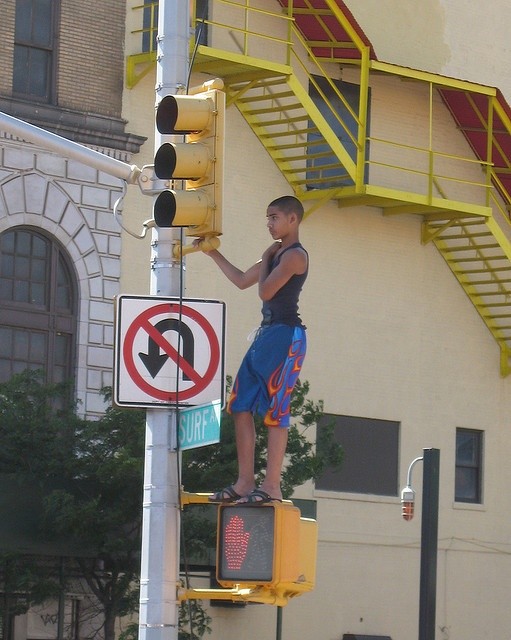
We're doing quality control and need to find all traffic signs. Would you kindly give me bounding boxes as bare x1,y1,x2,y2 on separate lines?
170,398,221,453
113,295,228,412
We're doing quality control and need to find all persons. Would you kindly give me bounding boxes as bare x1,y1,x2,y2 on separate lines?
193,195,309,505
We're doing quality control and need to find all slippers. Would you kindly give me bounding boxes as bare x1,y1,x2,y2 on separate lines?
235,489,282,506
208,487,243,503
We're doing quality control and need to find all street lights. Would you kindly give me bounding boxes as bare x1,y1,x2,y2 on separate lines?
399,446,445,640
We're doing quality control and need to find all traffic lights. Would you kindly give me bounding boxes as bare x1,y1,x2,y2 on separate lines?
209,496,319,596
145,78,223,238
